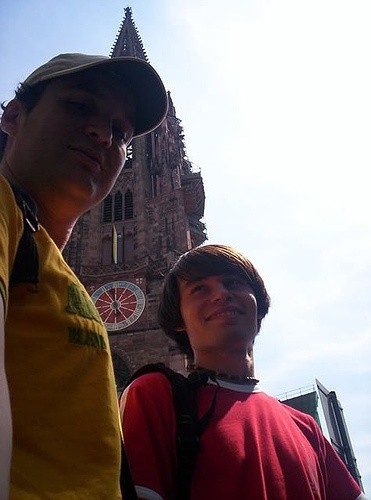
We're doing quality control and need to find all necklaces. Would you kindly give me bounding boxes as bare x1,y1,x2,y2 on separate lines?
186,363,259,385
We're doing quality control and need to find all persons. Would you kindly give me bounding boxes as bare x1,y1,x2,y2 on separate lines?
118,244,369,500
0,53,168,500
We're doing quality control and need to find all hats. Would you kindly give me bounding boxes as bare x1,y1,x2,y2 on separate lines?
15,53,169,139
